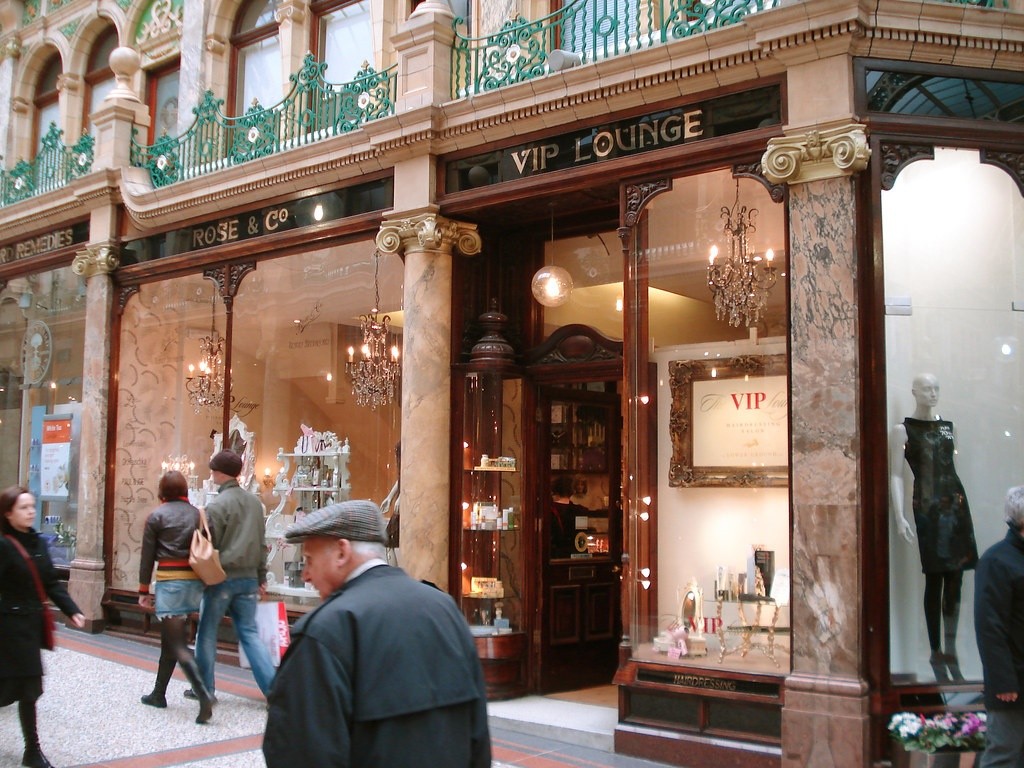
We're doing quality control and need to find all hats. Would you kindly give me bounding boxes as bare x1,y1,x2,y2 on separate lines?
209,449,243,477
285,499,388,544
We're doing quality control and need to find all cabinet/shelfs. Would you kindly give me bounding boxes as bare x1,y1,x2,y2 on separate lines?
551,468,608,518
257,431,352,589
463,469,524,601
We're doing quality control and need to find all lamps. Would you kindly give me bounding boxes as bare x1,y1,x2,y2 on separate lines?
530,212,572,311
176,281,233,413
706,163,783,340
548,49,580,70
343,242,400,408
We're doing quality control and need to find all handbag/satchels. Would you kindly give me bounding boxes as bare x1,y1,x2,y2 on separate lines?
189,508,227,587
38,607,54,650
239,591,292,668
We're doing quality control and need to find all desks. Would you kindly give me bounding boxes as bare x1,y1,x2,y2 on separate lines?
710,593,786,662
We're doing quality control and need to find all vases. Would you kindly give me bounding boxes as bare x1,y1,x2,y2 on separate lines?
891,739,982,767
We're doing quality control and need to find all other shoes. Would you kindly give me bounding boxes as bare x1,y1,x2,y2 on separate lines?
183,688,196,698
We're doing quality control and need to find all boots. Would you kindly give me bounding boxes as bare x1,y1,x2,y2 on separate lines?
181,659,217,723
20,698,53,768
141,657,177,709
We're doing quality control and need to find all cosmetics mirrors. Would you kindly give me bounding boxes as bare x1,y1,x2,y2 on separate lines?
676,581,705,654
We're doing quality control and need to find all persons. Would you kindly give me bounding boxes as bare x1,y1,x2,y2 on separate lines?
890,375,978,680
0,486,86,768
974,487,1024,768
183,451,275,710
381,441,400,569
553,477,587,558
137,471,218,723
261,508,492,768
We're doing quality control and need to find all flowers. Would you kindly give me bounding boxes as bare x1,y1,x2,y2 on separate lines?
887,710,990,751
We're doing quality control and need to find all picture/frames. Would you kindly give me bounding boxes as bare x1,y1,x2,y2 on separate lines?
669,354,788,490
